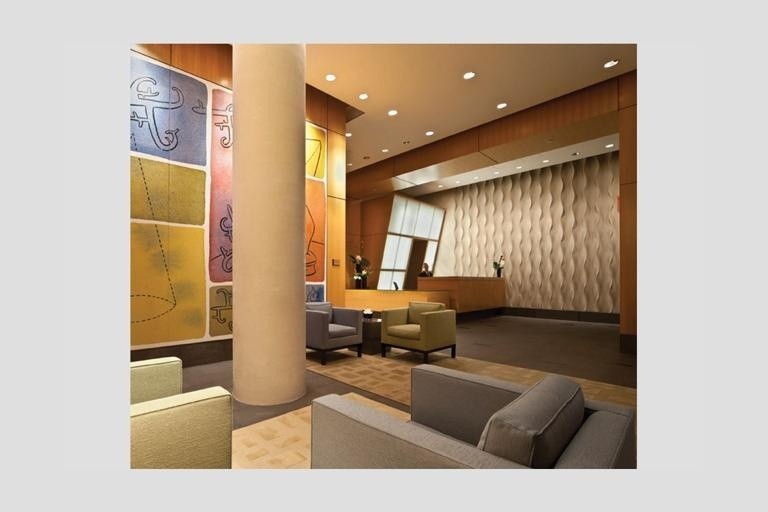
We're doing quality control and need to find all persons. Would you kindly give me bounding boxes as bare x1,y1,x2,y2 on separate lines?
417,263,433,276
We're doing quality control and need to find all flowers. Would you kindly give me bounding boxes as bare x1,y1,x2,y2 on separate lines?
493,255,506,270
348,251,375,279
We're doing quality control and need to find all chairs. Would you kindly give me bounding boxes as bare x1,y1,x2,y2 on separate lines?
299,299,456,367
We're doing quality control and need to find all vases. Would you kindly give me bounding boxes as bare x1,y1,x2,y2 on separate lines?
355,280,367,289
497,270,502,277
